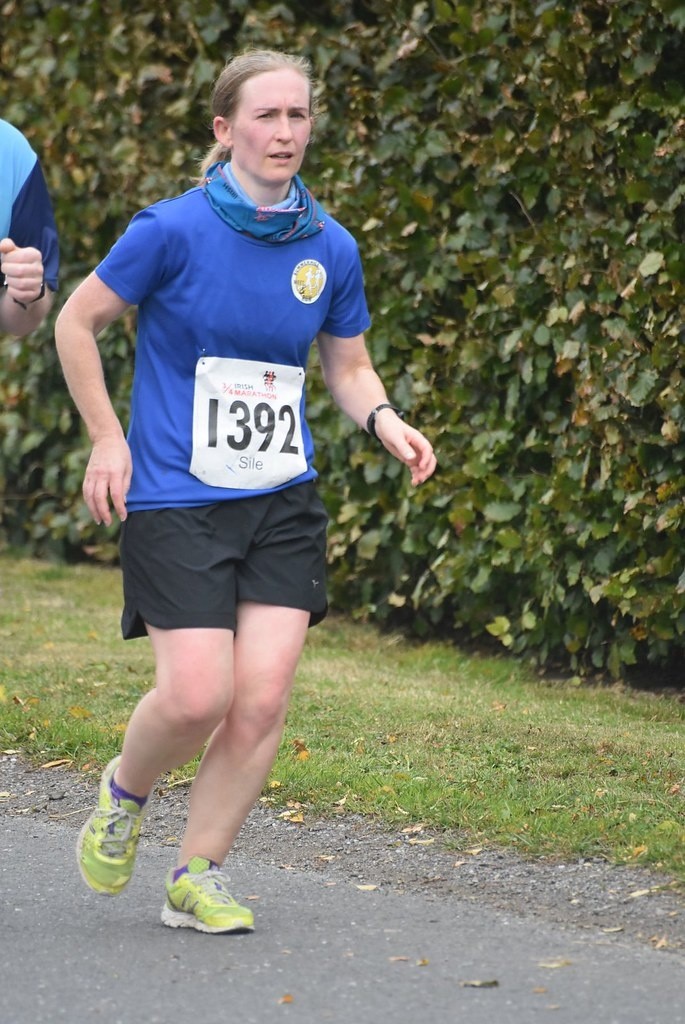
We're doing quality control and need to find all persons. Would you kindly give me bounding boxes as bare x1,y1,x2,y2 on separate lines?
0,119,60,337
53,51,437,933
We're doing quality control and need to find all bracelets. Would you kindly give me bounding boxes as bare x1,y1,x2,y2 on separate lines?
367,404,404,443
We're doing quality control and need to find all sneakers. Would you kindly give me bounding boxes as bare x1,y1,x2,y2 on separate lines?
76,755,152,898
161,857,255,933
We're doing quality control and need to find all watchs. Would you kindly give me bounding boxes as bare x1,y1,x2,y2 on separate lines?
3,276,45,309
